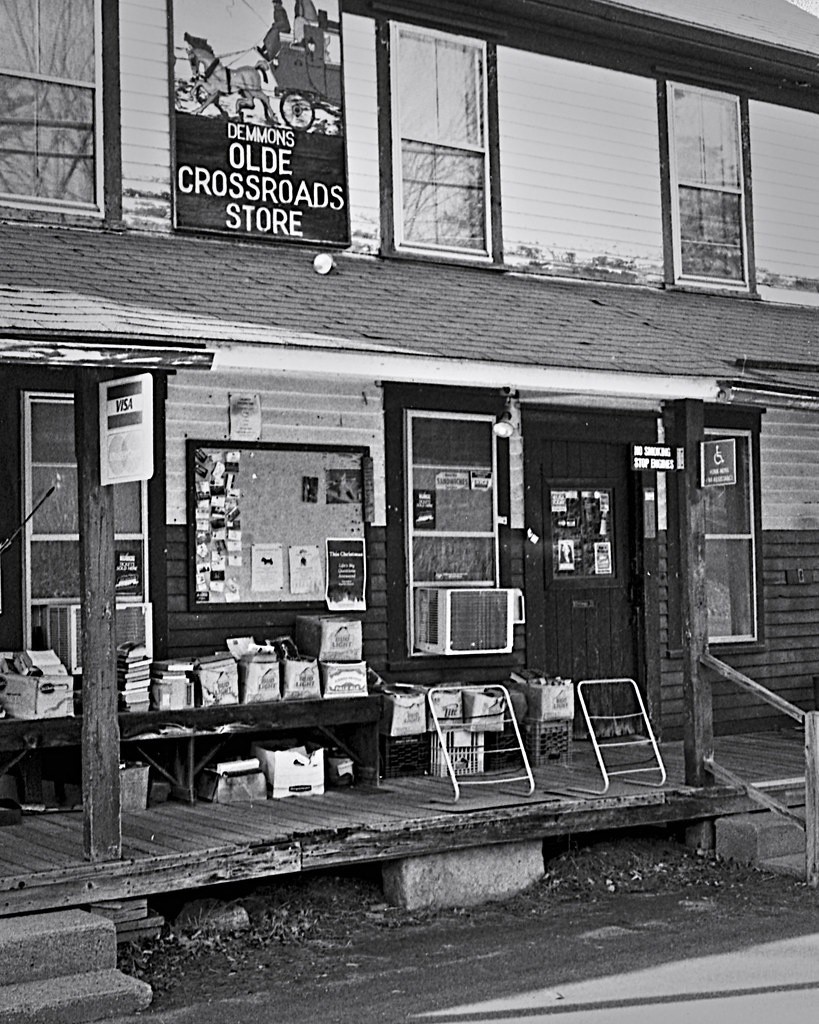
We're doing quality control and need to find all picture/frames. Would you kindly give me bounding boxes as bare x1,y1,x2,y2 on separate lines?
545,478,623,590
185,438,373,612
167,1,352,247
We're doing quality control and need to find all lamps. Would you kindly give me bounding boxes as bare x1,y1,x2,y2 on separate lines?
492,411,514,438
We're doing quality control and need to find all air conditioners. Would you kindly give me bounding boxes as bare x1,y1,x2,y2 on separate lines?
414,589,525,655
46,602,155,675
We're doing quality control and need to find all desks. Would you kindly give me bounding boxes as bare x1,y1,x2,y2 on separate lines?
0,694,379,805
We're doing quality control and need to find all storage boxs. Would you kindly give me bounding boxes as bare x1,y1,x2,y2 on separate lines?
280,655,322,701
196,758,268,804
193,663,239,707
295,614,362,662
431,731,484,777
485,722,526,770
320,661,369,700
0,650,74,720
519,720,573,767
152,682,194,711
251,738,325,799
379,693,426,737
379,731,430,778
464,691,505,731
503,672,574,721
119,760,151,812
411,685,463,732
238,663,281,704
